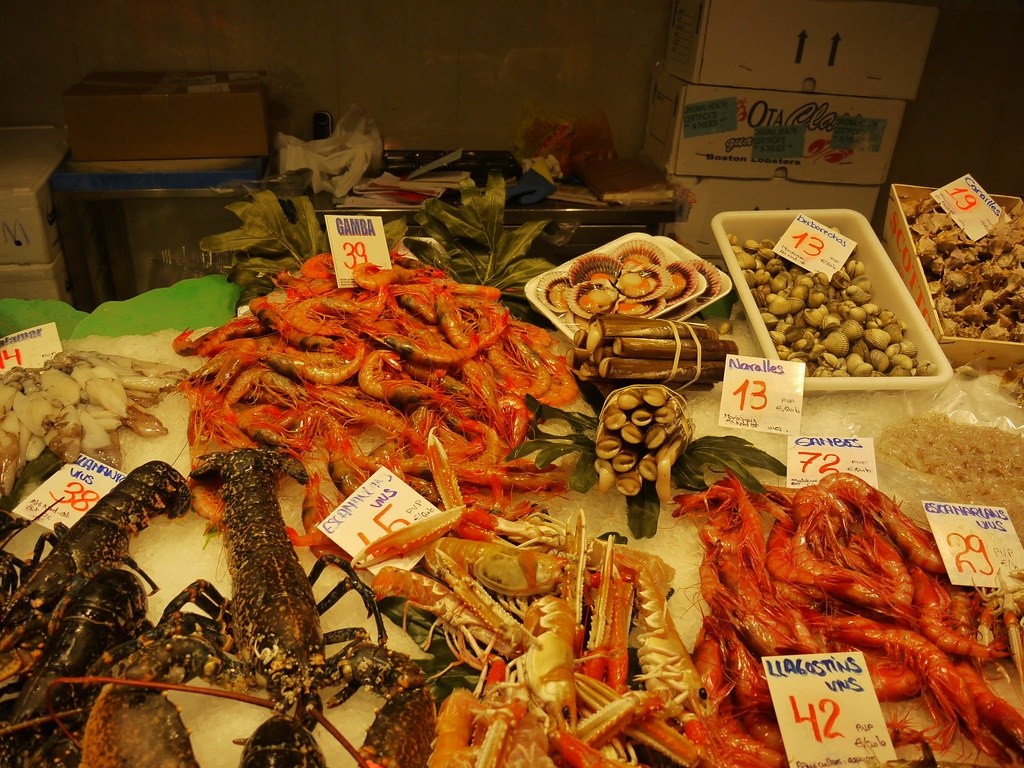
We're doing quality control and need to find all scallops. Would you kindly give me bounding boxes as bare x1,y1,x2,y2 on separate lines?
537,240,722,335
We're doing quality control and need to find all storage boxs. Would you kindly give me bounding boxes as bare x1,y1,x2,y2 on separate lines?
0,124,71,305
63,71,271,160
883,183,1024,369
644,0,939,271
710,207,954,397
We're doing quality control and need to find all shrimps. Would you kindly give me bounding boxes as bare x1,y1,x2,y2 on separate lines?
179,254,1024,768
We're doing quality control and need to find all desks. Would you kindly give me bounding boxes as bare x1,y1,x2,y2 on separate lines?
309,168,683,265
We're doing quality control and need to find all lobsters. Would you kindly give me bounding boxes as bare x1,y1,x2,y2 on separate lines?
0,444,438,767
